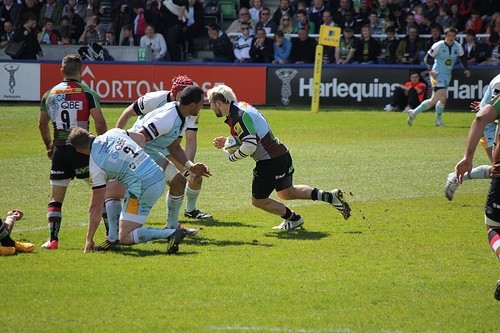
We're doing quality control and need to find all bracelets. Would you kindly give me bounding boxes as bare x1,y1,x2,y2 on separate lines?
185,160,194,170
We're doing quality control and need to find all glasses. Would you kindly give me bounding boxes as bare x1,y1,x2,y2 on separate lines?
242,28,248,31
261,12,269,15
240,12,248,16
282,17,291,22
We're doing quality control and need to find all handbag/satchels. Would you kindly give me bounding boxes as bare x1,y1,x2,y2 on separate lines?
5,40,28,57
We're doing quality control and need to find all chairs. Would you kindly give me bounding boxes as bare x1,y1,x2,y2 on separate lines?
186,0,240,57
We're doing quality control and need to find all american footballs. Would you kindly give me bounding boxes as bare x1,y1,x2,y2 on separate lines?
224,135,242,155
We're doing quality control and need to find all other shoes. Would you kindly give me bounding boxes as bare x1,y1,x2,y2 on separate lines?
494,279,500,300
384,104,399,111
403,106,411,112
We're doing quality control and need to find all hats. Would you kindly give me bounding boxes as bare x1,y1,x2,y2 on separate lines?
171,76,192,96
489,33,498,42
463,28,476,36
428,22,445,34
344,27,354,30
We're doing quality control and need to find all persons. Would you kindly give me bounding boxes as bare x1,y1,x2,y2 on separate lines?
383,70,426,112
445,74,500,302
0,0,500,65
207,85,351,231
407,27,470,127
69,127,189,254
39,55,108,249
128,86,212,236
116,75,213,220
0,209,35,256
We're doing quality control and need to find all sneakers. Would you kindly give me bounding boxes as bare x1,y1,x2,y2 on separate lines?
178,225,199,236
96,238,120,251
327,188,353,219
444,171,459,201
41,238,59,251
14,241,36,252
166,229,187,255
0,246,16,255
184,209,213,220
435,120,448,128
408,109,414,126
272,215,305,232
